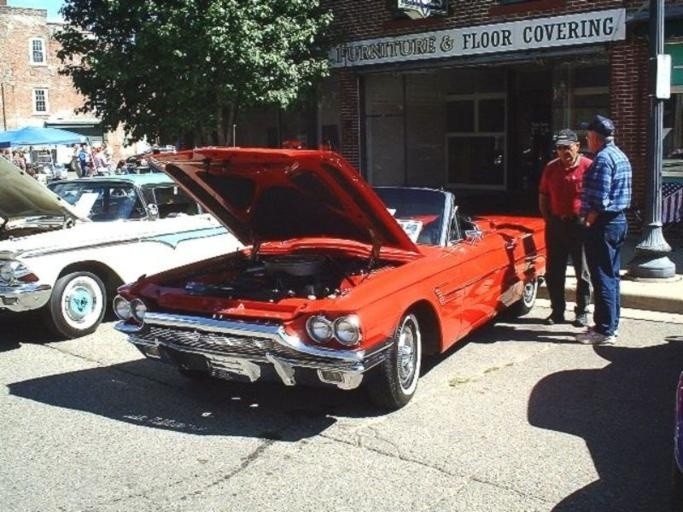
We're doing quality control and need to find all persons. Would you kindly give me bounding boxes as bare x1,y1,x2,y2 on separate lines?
575,115,634,346
0,138,129,187
536,129,594,327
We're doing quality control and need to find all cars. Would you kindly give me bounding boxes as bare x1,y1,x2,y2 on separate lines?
112,145,548,411
0,152,246,340
117,145,176,174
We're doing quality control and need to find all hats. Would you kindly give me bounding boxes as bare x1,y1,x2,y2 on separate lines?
556,129,576,145
581,115,615,136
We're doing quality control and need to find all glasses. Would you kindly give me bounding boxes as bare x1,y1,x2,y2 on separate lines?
556,143,576,150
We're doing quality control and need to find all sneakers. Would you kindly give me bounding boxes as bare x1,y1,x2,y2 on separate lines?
545,311,588,326
576,325,620,344
